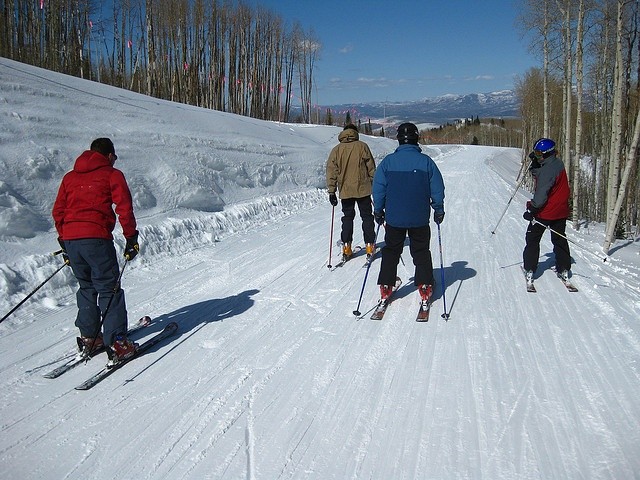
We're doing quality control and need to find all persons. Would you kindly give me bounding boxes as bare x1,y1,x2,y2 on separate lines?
523,137,572,281
325,124,376,260
52,138,140,362
372,122,446,301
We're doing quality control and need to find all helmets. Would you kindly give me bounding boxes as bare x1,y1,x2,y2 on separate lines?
397,123,419,145
533,138,556,159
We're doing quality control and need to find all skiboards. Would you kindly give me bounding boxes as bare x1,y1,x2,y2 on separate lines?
521,264,580,292
43,315,178,390
329,246,379,268
368,275,433,322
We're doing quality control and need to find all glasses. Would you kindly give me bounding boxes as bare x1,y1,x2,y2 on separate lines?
111,154,118,161
534,153,544,159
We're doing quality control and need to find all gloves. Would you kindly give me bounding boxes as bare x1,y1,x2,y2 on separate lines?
124,230,140,260
523,205,539,221
329,193,338,206
53,237,71,266
375,212,385,224
529,151,541,168
434,212,445,223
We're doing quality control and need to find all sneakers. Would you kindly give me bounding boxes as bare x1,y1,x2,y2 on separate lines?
380,284,392,301
343,242,353,258
527,270,534,283
561,271,570,280
366,243,376,257
82,333,105,351
419,284,433,302
109,336,140,360
